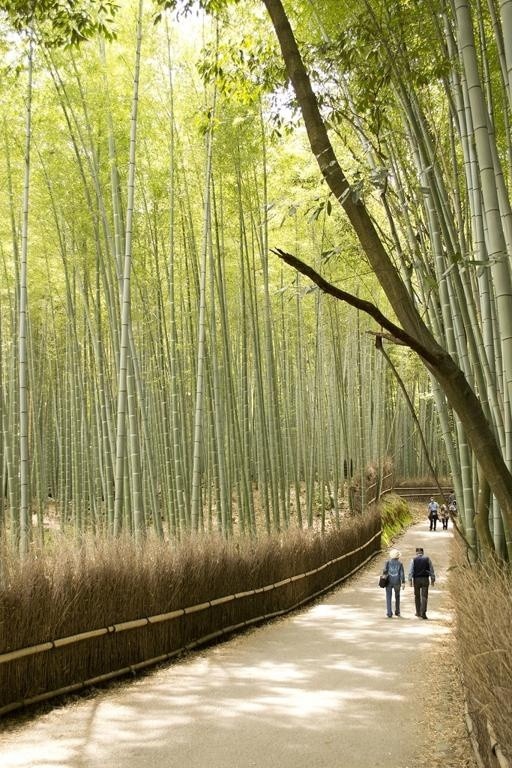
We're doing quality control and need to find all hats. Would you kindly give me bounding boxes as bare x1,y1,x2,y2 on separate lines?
388,548,401,559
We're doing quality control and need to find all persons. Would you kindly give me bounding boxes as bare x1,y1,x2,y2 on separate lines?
446,489,455,509
426,497,440,532
438,497,449,530
407,547,435,620
381,548,406,617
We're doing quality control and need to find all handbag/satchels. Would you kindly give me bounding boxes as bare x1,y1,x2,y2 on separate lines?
378,575,390,588
